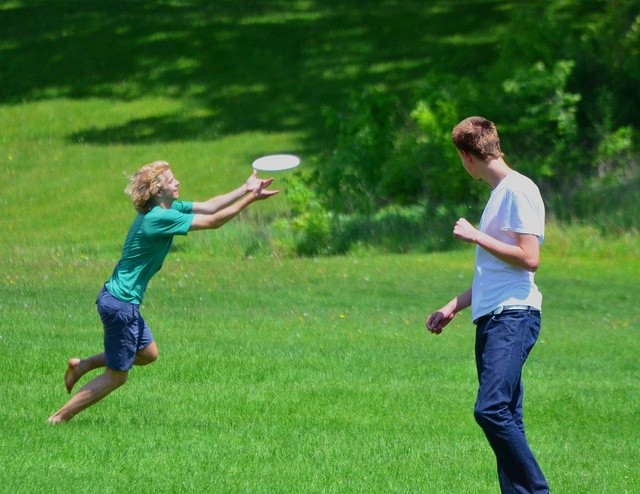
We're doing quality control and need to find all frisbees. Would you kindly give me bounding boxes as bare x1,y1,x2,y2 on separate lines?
253,154,300,171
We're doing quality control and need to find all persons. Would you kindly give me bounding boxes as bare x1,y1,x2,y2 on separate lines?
49,161,281,426
427,117,549,493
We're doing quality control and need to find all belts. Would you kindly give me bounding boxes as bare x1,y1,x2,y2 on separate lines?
487,305,540,315
